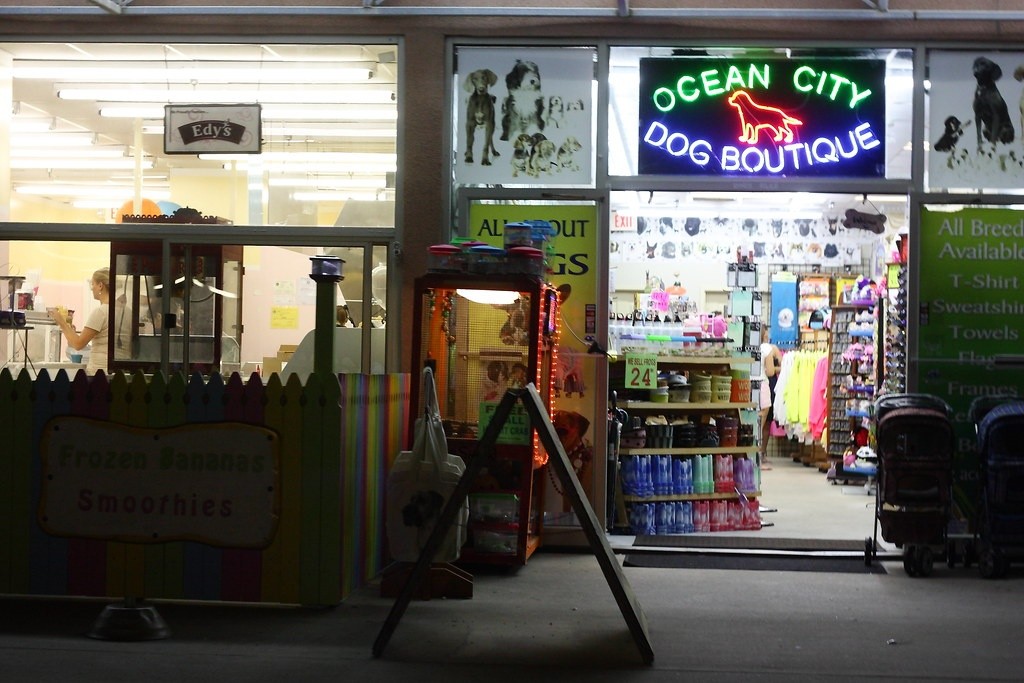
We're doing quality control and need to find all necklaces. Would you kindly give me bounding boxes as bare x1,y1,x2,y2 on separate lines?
547,461,585,498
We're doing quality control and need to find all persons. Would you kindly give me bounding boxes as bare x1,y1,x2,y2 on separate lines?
139,299,183,335
759,324,782,470
536,412,593,525
53,267,136,374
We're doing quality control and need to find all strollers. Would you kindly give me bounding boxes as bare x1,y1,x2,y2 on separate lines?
960,395,1024,579
864,394,957,577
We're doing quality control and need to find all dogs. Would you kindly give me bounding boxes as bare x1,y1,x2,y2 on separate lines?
460,57,586,180
463,284,595,513
932,53,1023,181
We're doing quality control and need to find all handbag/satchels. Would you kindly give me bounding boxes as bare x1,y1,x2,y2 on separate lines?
386,370,471,563
